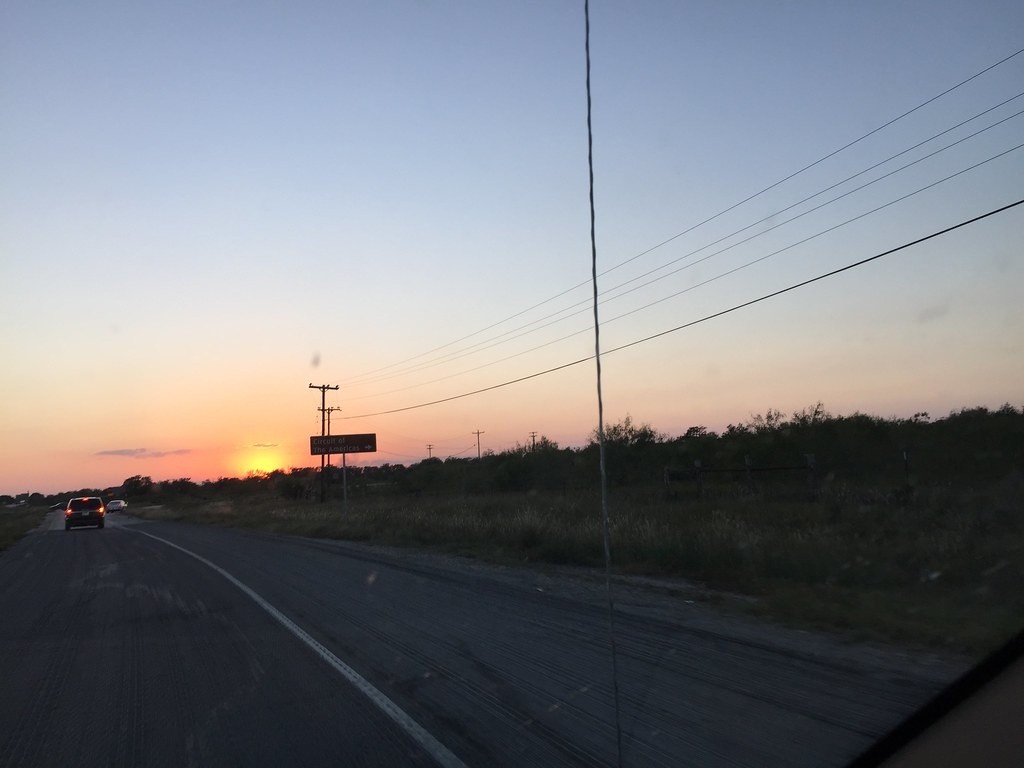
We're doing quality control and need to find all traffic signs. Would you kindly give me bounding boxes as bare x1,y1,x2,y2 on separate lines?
310,433,377,456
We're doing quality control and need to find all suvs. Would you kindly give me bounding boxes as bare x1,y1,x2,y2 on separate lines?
64,496,106,531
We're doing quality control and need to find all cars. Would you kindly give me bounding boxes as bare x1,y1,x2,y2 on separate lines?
106,499,128,514
